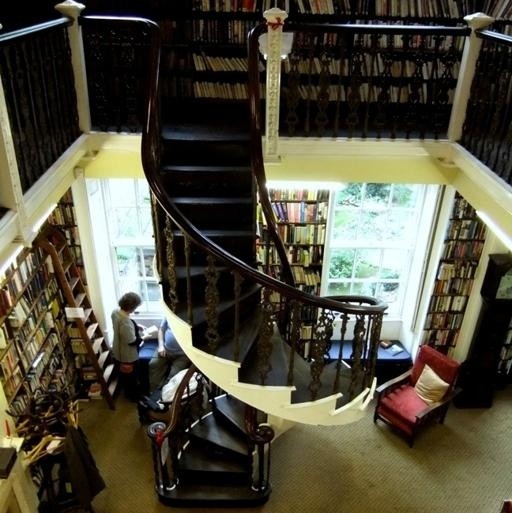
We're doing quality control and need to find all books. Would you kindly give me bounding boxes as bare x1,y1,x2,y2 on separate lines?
256,204,327,223
197,0,512,17
258,266,320,288
499,322,511,378
261,290,318,340
436,263,477,278
0,322,32,431
192,18,512,51
2,247,50,321
186,53,512,78
35,323,97,414
256,186,329,203
441,242,484,261
422,314,460,330
450,191,478,219
188,81,510,105
434,280,473,296
53,194,90,321
428,298,466,311
419,331,458,347
258,248,321,264
446,222,488,239
258,225,325,244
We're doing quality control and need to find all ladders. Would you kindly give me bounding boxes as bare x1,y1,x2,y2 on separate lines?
36,222,125,411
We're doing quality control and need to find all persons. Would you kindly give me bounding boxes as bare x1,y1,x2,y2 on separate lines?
109,292,146,399
148,319,188,396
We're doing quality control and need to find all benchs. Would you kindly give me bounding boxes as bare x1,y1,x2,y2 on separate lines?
139,336,411,393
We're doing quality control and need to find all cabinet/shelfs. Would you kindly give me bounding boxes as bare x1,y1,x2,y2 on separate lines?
1,187,107,444
411,193,486,358
454,253,512,410
163,0,466,138
257,188,332,328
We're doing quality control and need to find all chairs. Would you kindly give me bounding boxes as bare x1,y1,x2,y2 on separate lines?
374,344,461,448
